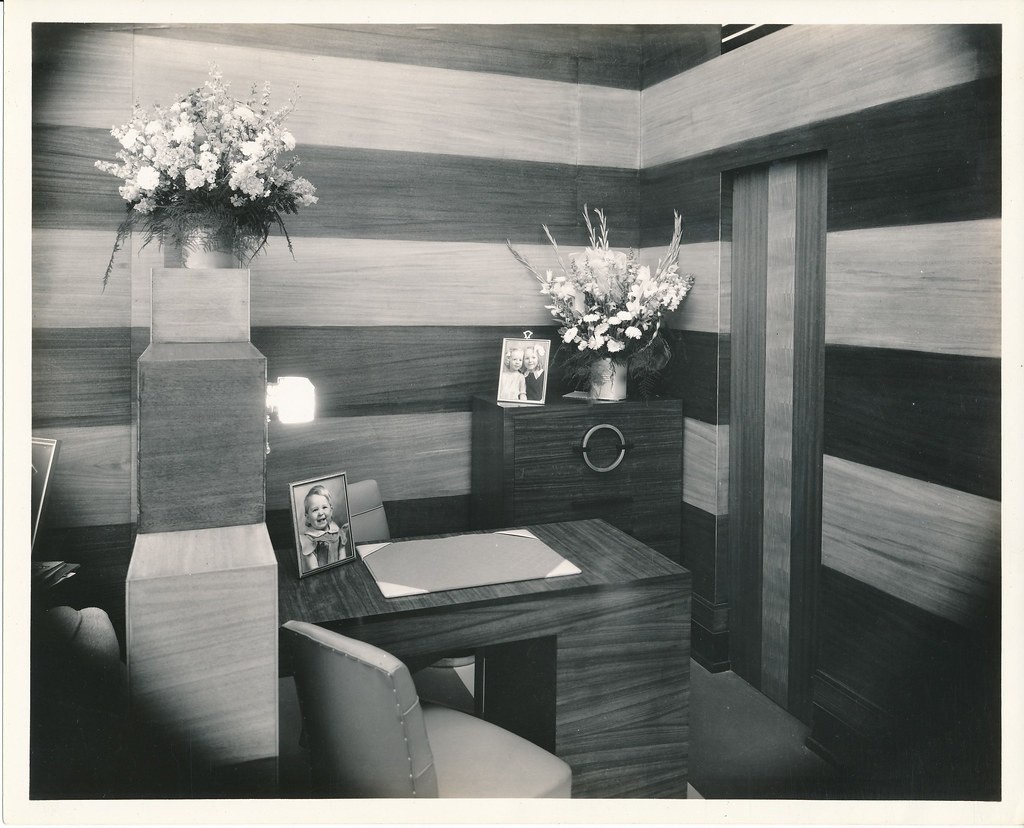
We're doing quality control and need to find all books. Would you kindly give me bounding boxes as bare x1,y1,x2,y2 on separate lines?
32,560,81,591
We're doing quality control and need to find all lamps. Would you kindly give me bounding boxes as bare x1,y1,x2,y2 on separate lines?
264,375,317,426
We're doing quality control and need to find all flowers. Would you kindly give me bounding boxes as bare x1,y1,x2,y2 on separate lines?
506,203,696,405
102,63,318,297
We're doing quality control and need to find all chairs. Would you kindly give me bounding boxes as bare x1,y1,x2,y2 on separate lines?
346,479,391,544
280,620,572,798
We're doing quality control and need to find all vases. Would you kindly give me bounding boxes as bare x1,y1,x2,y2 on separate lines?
589,358,628,401
179,211,235,268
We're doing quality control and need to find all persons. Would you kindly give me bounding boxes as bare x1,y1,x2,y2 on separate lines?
298,486,349,573
499,346,545,402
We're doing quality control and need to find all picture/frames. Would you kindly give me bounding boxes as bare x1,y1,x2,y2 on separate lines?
497,336,551,404
290,471,358,578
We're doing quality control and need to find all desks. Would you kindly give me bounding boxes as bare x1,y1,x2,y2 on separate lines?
272,518,690,799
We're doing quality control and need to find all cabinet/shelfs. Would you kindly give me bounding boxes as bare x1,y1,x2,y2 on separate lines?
470,389,684,568
